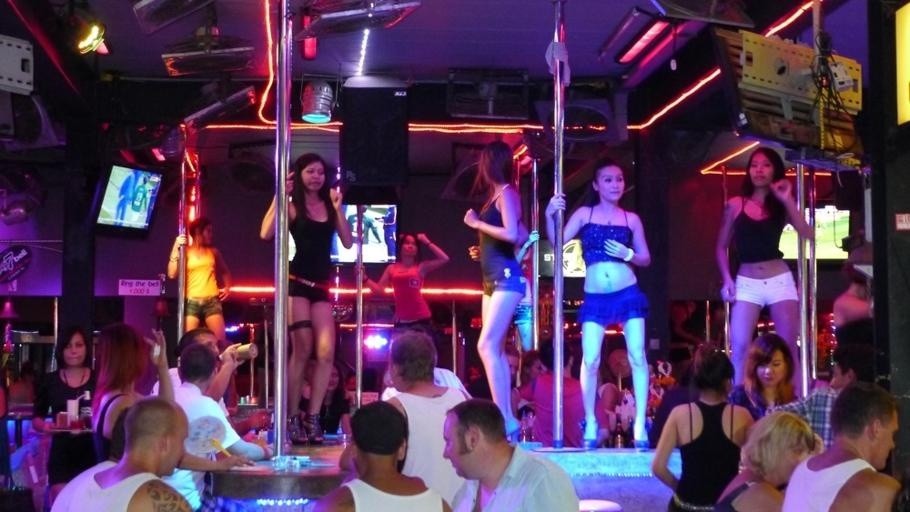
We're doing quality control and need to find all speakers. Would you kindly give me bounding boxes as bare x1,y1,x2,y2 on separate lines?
340,88,410,183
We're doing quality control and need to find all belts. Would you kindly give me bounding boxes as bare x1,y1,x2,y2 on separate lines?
672,493,716,511
396,319,418,324
288,274,326,290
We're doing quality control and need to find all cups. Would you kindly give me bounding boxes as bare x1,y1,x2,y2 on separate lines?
57,398,91,431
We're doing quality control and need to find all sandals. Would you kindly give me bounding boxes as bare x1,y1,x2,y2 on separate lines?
287,414,309,447
302,413,325,447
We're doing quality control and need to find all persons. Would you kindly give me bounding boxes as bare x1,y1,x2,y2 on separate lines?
373,205,396,262
541,157,661,452
463,135,528,441
830,256,879,343
114,169,160,227
328,205,347,263
710,143,818,403
166,213,241,417
256,148,355,448
347,204,382,263
512,227,544,392
355,226,450,373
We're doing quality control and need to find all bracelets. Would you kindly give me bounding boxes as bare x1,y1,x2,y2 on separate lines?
622,247,635,262
168,253,180,263
424,239,434,248
365,276,369,284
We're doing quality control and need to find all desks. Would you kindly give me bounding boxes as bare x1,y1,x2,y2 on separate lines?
210,444,353,511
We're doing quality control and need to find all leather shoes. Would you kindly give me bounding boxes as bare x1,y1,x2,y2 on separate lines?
633,432,651,451
582,435,598,449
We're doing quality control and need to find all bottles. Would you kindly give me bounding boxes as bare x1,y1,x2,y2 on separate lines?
219,342,258,362
613,412,626,448
519,409,532,441
240,395,258,404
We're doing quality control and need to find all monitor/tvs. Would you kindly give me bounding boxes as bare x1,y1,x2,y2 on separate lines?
85,161,163,239
709,25,865,158
538,237,588,279
329,203,400,265
778,208,851,265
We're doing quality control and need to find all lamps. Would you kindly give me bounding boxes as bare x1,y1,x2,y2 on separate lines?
298,71,341,124
46,0,108,57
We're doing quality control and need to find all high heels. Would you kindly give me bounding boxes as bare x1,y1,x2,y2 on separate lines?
501,416,523,445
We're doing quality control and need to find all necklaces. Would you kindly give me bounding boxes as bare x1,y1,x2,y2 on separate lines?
597,204,619,226
